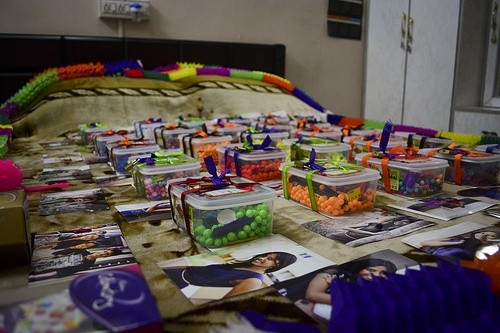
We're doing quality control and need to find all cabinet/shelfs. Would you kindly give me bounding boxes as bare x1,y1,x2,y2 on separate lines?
362,1,500,142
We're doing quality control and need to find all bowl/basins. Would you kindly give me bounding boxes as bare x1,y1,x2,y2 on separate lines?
77,113,500,249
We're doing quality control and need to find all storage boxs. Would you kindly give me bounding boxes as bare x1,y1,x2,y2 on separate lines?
79,112,499,249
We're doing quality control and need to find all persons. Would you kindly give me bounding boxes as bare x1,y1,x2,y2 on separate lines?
164,252,297,300
30,235,121,280
420,231,500,263
280,259,397,306
325,215,412,243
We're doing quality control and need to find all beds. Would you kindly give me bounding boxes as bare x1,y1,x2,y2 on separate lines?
0,33,499,333
